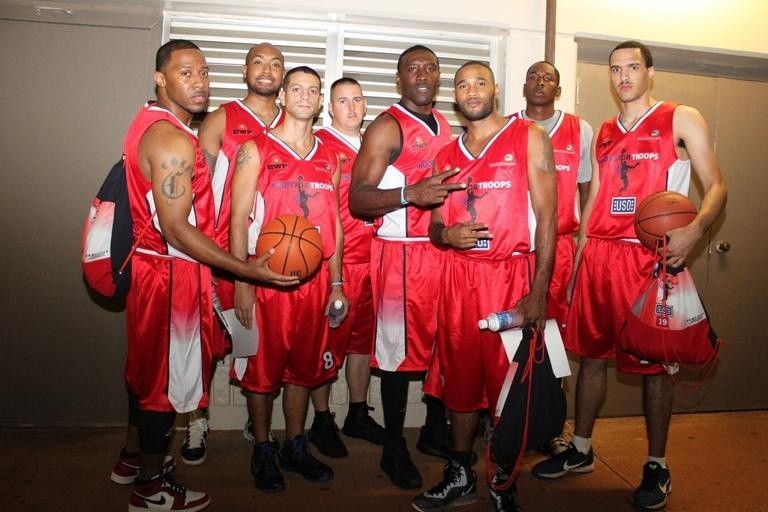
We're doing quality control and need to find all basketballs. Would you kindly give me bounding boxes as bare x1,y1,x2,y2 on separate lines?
634,192,698,251
256,215,322,283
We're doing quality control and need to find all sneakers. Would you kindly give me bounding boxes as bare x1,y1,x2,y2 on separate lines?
279,436,334,483
487,469,522,512
307,410,348,458
532,442,595,478
243,421,280,454
634,461,673,510
180,418,210,466
411,456,478,512
343,402,386,444
127,474,211,512
251,441,286,494
381,439,422,489
111,447,176,484
546,435,569,458
417,425,478,466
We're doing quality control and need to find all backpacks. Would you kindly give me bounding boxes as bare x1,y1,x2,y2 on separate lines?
81,120,196,314
619,233,711,390
484,325,567,492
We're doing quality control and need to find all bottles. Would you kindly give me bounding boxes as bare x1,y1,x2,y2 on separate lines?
478,309,526,331
328,299,345,328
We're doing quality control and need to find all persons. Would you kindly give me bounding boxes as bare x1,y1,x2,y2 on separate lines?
231,67,347,492
178,42,281,466
348,46,478,490
109,38,301,512
309,77,390,458
531,41,728,510
411,61,558,511
502,63,595,460
